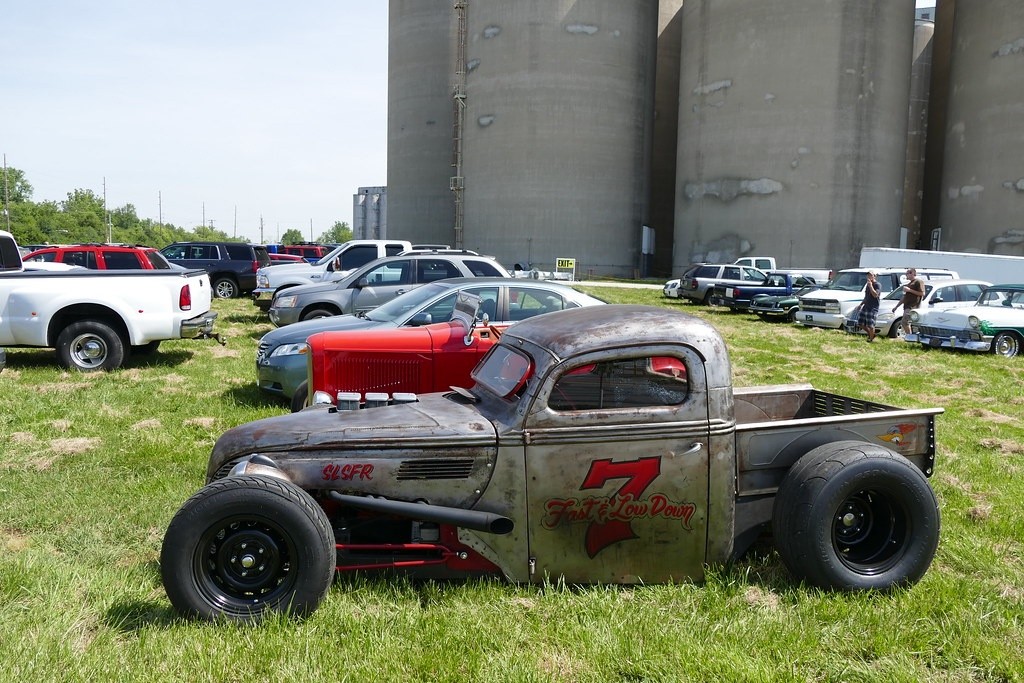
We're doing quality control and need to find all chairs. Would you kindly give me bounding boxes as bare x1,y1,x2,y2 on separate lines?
769,279,776,286
859,278,867,286
537,296,566,314
418,266,427,283
778,279,784,286
943,291,954,302
477,299,496,321
400,263,409,284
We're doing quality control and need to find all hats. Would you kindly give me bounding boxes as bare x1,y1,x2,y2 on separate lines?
867,269,877,275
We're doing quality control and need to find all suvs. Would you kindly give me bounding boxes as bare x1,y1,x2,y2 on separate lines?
20,241,332,300
266,250,522,328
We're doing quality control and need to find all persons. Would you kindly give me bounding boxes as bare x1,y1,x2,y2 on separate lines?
892,268,925,339
857,271,882,342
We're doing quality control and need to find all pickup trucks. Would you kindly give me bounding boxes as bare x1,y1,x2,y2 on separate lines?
158,306,948,634
732,256,833,287
0,229,228,373
712,272,824,314
252,239,483,312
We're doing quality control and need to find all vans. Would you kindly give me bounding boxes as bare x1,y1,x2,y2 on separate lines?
793,267,960,330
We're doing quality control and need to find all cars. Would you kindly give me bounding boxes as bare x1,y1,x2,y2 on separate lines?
748,284,825,324
842,278,1006,339
904,283,1024,357
662,279,681,299
676,262,782,306
255,276,607,402
306,289,686,409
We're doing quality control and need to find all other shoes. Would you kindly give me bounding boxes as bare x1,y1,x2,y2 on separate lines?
867,333,877,342
